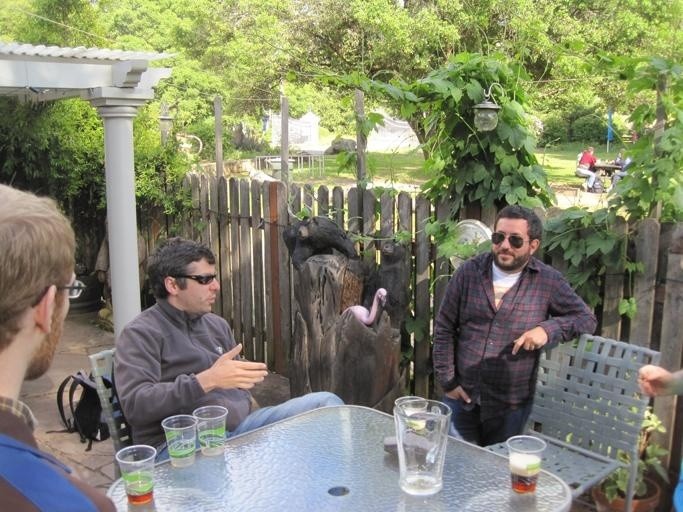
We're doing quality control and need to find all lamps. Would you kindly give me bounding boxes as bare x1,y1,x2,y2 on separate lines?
471,81,504,133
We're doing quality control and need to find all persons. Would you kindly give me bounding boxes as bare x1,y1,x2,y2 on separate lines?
433,206,598,446
114,237,345,466
0,184,116,512
638,365,683,512
576,147,632,192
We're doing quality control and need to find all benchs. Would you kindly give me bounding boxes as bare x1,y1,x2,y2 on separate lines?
621,134,636,143
574,172,588,180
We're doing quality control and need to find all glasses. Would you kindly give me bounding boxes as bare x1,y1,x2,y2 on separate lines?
57,280,86,301
167,270,217,287
490,232,537,249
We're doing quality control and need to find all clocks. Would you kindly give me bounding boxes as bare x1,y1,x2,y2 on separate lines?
447,219,495,272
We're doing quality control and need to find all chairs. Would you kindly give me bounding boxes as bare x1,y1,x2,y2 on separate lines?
480,333,662,512
89,348,133,483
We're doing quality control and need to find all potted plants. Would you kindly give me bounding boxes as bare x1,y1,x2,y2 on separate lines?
529,355,674,511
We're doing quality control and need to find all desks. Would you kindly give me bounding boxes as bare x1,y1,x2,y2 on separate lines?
590,164,622,180
106,405,574,512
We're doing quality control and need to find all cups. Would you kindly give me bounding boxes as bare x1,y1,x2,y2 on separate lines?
392,400,452,496
395,395,428,434
191,405,227,457
115,444,155,504
504,435,546,496
160,414,198,467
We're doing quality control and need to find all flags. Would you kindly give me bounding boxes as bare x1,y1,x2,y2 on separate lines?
608,111,613,140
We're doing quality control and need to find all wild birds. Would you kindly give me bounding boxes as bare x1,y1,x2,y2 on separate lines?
342,287,387,326
